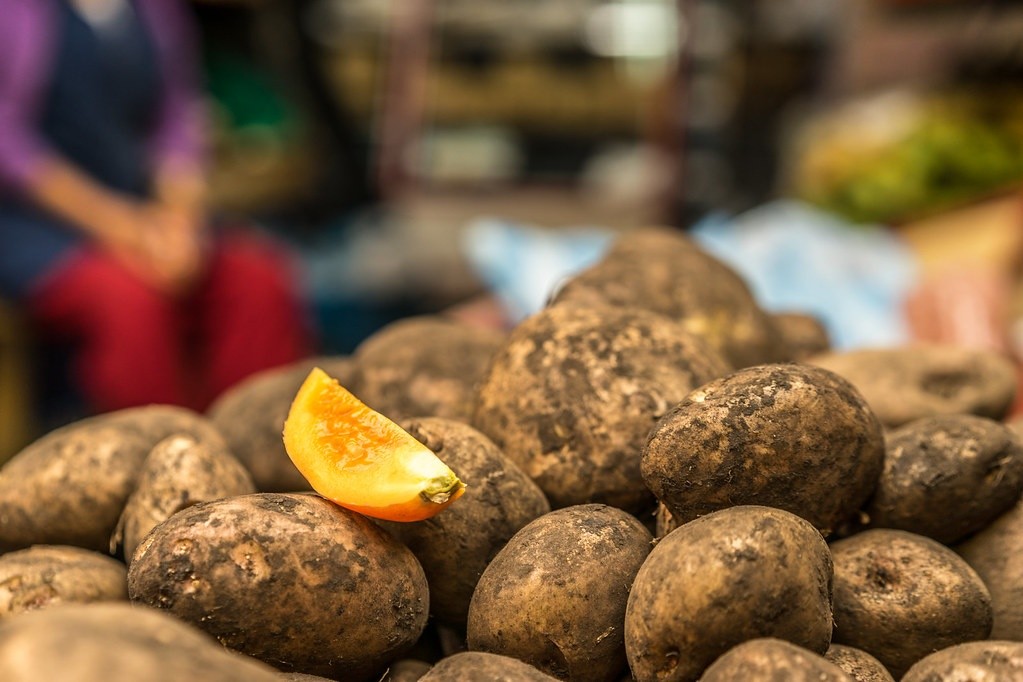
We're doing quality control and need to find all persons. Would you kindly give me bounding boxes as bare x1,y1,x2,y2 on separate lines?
1,0,306,425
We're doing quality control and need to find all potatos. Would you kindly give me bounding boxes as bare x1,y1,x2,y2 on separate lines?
0,224,1023,678
